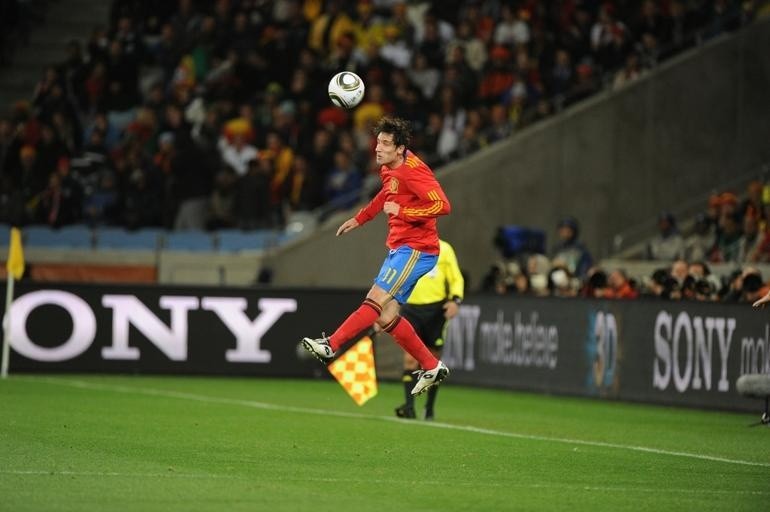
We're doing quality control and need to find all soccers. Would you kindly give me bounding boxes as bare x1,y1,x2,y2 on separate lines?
329,71,365,109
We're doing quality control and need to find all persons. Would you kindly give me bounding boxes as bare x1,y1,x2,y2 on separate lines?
299,113,451,397
393,235,464,419
751,289,770,424
0,0,761,233
476,178,769,306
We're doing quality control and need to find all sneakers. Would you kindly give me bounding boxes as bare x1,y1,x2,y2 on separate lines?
301,331,336,366
395,404,416,419
410,360,450,398
424,408,433,419
